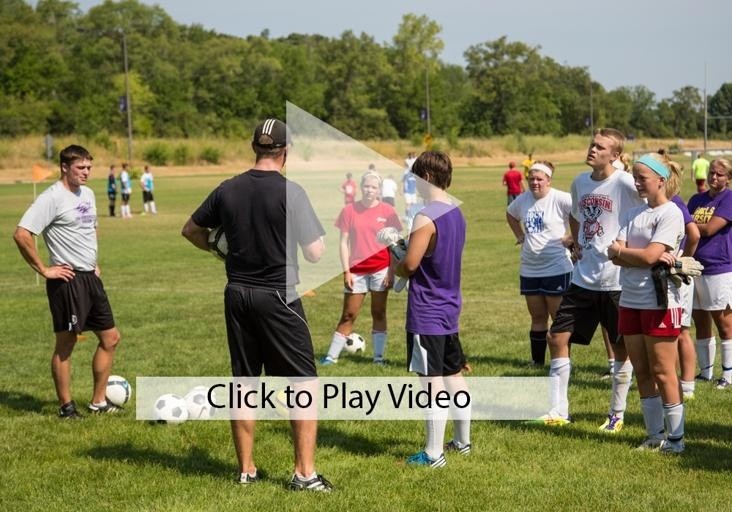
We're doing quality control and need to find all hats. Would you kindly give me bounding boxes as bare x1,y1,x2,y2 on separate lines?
253,119,294,149
509,160,516,167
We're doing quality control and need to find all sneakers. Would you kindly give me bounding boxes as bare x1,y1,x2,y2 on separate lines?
60,407,82,420
599,416,624,434
405,451,446,469
320,357,338,366
660,438,684,454
374,358,384,366
523,409,570,428
601,372,612,379
443,440,470,456
682,390,694,401
238,470,263,484
289,474,334,493
716,378,731,390
635,438,665,454
88,403,124,413
694,374,715,384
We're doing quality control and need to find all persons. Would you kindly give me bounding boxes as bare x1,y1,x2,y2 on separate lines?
524,153,534,179
662,160,702,406
505,162,523,208
108,165,118,216
692,153,711,192
342,173,356,205
607,153,703,455
139,166,155,216
182,117,334,492
379,176,396,207
505,161,572,368
685,159,732,388
379,152,471,468
405,153,418,212
120,163,132,219
13,145,120,422
320,171,402,364
519,129,645,431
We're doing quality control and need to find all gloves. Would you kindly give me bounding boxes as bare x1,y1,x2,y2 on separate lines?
667,266,692,287
669,257,704,276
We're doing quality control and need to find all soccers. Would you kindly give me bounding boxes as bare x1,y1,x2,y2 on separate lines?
185,385,216,421
154,394,189,426
105,375,132,407
208,227,228,259
344,332,367,355
